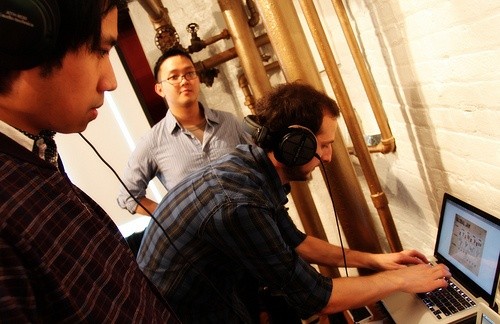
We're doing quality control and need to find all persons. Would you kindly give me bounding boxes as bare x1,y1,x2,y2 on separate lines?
116,47,255,217
0,0,184,324
136,81,452,324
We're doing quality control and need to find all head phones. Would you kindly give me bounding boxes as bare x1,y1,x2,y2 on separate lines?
241,115,318,167
0,0,58,70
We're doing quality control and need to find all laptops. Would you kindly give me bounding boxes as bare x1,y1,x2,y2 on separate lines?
476,302,500,324
380,192,500,324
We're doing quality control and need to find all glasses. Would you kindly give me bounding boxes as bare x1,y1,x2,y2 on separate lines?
157,71,197,86
